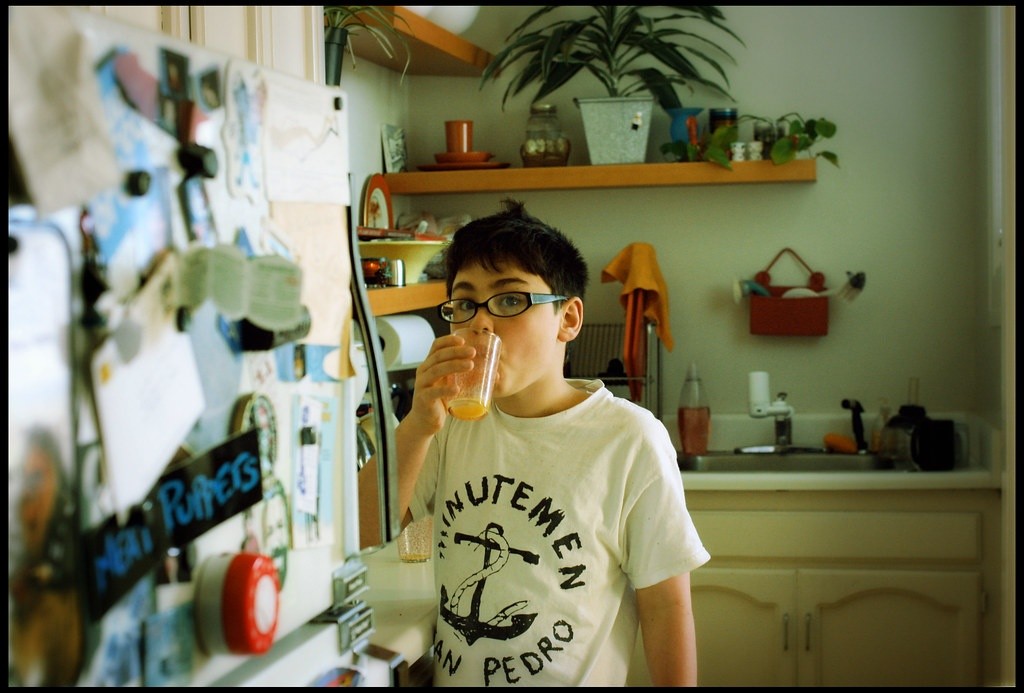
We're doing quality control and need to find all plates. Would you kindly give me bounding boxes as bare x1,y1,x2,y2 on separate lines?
417,159,511,169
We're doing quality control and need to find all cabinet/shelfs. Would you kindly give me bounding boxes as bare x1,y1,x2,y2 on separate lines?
628,510,985,687
323,5,816,318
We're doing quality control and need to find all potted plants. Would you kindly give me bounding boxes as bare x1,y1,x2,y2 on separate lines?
660,112,841,169
478,5,747,164
324,5,415,86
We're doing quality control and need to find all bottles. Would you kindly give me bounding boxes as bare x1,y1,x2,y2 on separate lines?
520,100,571,165
675,363,711,458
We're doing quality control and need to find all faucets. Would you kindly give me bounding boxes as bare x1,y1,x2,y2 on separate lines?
747,370,792,454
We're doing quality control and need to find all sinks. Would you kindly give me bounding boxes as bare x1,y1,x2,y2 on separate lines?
676,451,922,476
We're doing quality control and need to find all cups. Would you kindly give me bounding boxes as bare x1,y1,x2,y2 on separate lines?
912,416,964,470
443,328,501,421
445,121,473,152
396,515,435,564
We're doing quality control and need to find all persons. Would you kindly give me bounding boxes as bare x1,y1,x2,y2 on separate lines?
358,199,705,689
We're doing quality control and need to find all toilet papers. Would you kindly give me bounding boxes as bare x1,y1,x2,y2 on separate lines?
356,315,439,369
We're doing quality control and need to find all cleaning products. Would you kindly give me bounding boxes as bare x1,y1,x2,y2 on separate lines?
676,361,712,455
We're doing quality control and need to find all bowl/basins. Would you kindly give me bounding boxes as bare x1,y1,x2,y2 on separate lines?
434,152,491,162
357,242,447,284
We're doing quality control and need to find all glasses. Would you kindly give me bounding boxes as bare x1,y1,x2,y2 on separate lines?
437,291,569,325
